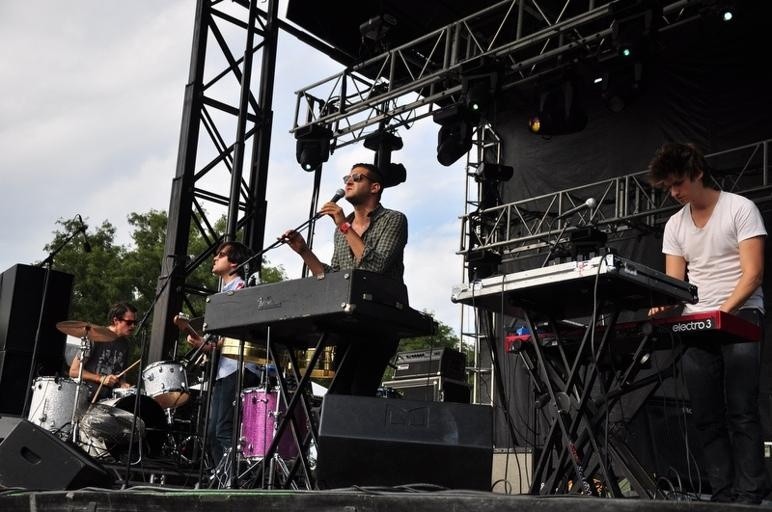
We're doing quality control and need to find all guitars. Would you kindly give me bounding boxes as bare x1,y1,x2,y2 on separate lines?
174,311,221,385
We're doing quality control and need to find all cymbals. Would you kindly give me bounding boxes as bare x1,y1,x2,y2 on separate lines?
56,319,119,343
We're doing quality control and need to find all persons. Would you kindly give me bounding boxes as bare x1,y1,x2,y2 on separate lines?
649,142,769,503
277,164,408,396
70,302,138,412
188,241,265,484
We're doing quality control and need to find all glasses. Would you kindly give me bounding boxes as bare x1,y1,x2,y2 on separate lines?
343,174,374,182
219,253,227,258
121,319,137,326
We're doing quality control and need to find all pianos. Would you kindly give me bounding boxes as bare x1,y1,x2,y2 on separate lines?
504,311,761,351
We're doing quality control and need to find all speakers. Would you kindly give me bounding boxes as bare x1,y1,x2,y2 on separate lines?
315,393,495,492
0,262,75,417
0,417,115,491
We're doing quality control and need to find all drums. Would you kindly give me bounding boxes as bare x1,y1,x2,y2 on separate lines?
113,387,145,400
27,375,94,445
222,336,272,366
240,385,310,464
78,393,167,463
142,359,190,408
286,346,338,379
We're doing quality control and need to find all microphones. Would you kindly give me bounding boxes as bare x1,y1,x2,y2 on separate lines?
76,217,92,253
559,198,596,216
329,188,347,203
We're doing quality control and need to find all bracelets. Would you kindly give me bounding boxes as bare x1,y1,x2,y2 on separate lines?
98,373,109,384
337,219,353,237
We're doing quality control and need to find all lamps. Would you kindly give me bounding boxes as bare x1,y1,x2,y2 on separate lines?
293,104,480,190
